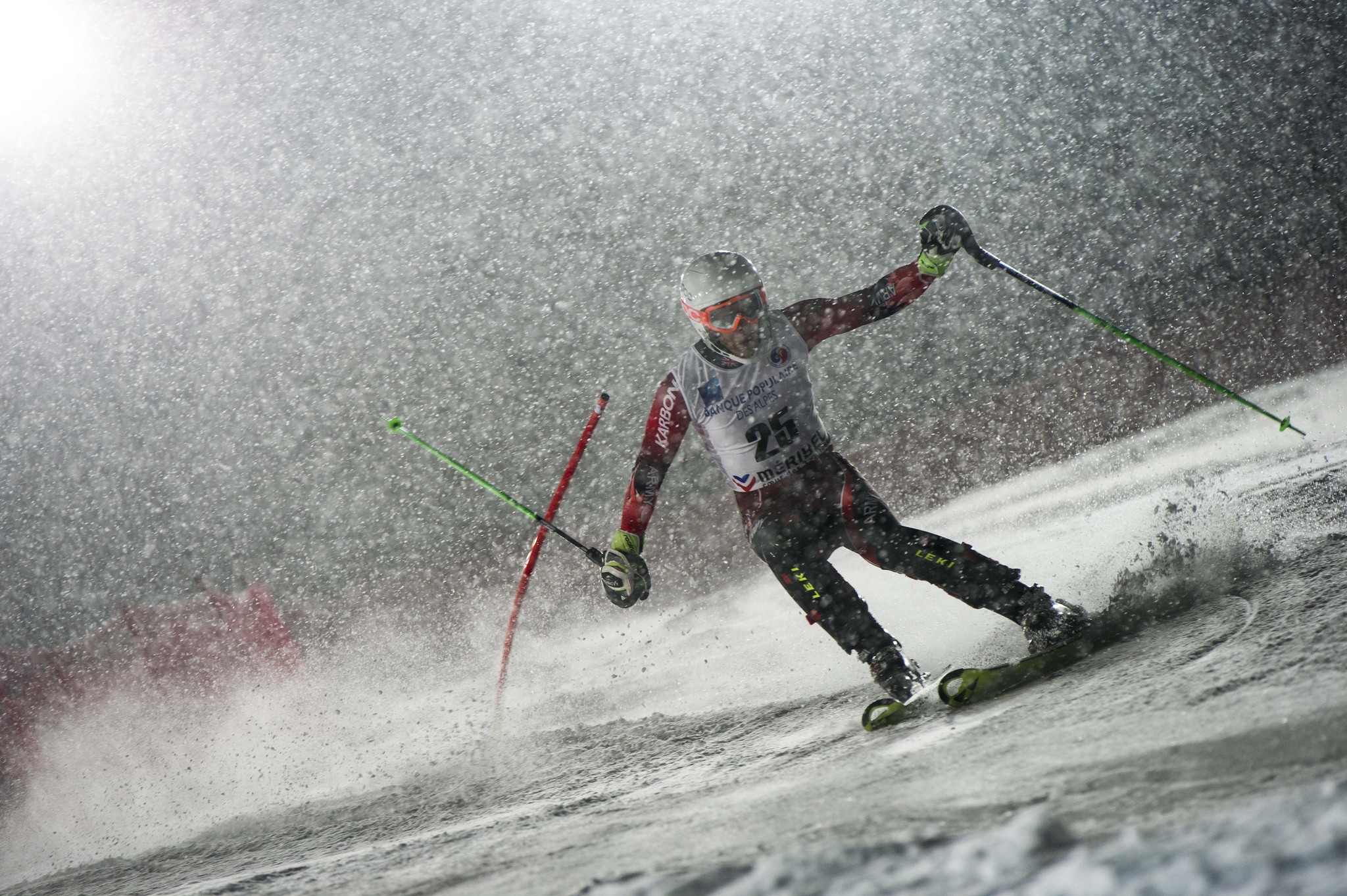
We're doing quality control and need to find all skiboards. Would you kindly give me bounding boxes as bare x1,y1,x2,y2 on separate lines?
860,623,1095,731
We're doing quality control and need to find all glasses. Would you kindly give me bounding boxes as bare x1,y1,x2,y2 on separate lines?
705,289,768,333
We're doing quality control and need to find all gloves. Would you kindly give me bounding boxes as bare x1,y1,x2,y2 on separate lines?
601,528,652,608
917,206,964,278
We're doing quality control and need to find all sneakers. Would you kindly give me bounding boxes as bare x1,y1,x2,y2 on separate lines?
1017,583,1088,655
869,644,924,702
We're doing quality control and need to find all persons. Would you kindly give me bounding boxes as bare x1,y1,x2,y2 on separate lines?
600,206,1086,709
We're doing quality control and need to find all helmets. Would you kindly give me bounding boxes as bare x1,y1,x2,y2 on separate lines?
681,251,769,365
